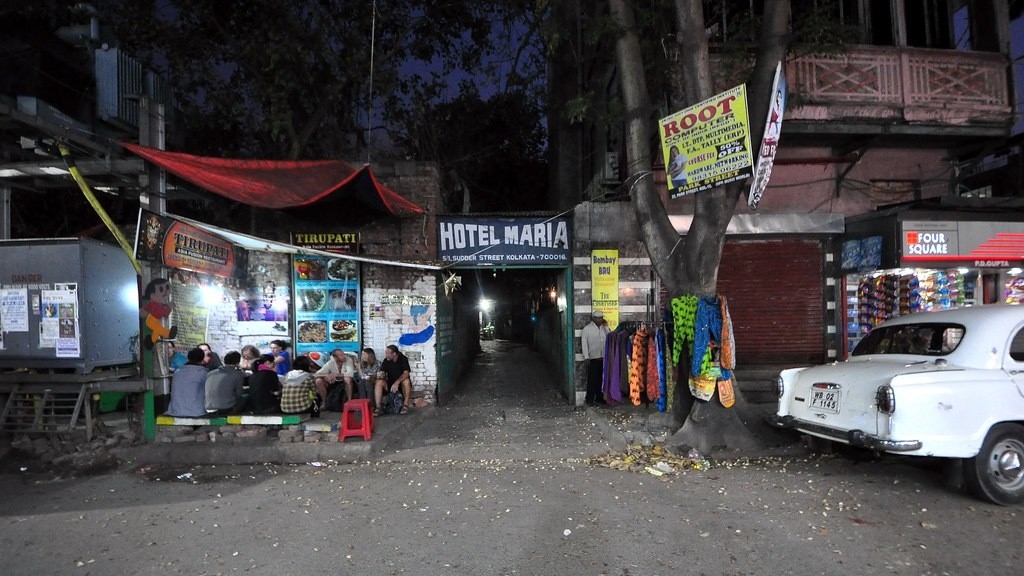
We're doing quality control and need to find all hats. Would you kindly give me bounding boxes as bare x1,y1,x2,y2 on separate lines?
592,311,606,317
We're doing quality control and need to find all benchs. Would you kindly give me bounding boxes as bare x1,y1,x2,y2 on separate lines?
156,413,310,426
891,333,928,355
397,389,424,399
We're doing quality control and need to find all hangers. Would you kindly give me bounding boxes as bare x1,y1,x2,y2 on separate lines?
618,321,666,334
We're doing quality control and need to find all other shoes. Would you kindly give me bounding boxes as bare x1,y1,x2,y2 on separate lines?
399,405,409,415
371,408,385,417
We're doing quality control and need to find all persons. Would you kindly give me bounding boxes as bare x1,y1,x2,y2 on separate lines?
204,351,249,418
356,348,382,411
775,91,784,134
280,356,323,418
666,146,688,188
247,353,282,415
168,341,187,373
241,345,260,369
184,343,223,372
581,311,612,406
268,340,291,376
372,345,412,417
314,349,358,411
163,348,209,418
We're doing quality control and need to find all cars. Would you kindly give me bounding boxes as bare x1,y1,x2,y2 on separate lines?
771,301,1024,506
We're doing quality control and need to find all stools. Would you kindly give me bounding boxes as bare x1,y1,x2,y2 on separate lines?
338,398,375,442
